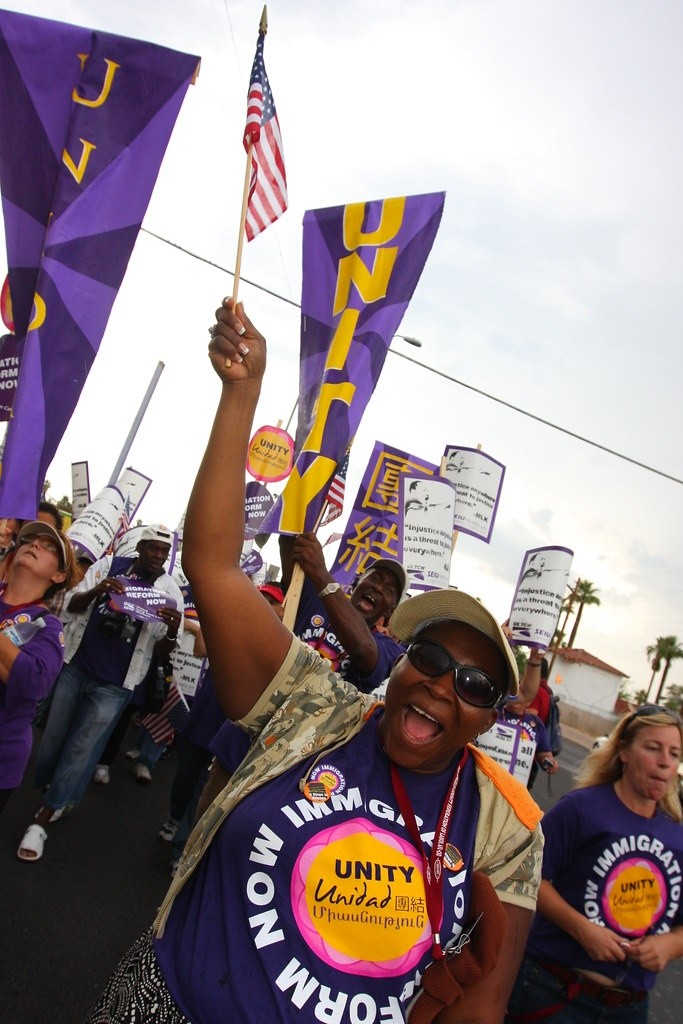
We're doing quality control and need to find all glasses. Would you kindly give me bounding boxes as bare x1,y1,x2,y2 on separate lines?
636,706,683,724
20,533,59,555
406,640,504,709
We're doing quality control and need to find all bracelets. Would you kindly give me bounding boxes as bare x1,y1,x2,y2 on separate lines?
526,659,542,667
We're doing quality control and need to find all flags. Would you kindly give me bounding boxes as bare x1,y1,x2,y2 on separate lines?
243,31,289,242
142,683,189,744
325,532,344,544
320,451,349,527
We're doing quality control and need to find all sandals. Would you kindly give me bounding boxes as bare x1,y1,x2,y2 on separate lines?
35,801,65,822
17,824,48,861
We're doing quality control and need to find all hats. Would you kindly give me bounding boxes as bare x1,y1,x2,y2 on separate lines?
364,558,411,606
134,524,173,549
17,521,72,570
388,589,519,697
259,581,285,603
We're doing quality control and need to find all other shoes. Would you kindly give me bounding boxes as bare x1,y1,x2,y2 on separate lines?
158,820,178,841
94,764,109,784
134,762,151,781
126,749,142,758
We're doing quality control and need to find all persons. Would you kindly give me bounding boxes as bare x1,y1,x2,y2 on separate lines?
0,499,410,878
502,702,683,1024
470,617,562,789
88,294,546,1024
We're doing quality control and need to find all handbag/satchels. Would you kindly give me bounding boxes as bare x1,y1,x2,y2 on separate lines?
543,693,562,756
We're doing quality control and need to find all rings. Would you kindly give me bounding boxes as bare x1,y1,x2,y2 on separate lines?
105,584,110,587
208,325,216,339
170,617,174,622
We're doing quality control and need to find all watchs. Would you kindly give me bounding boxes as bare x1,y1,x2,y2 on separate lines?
165,632,180,640
319,582,341,599
